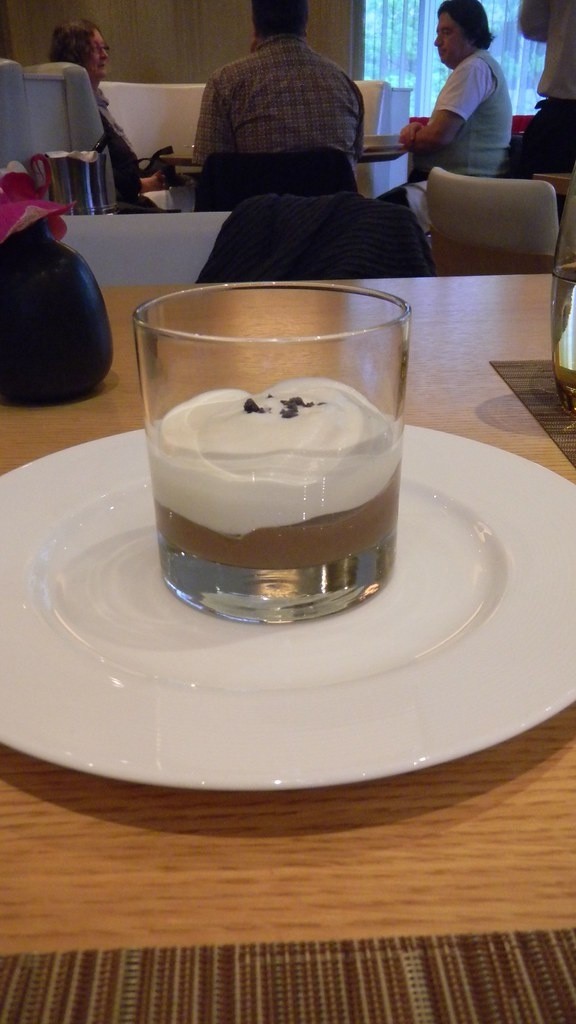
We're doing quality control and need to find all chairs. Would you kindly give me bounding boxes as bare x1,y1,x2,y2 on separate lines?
425,165,561,274
196,191,431,284
196,144,365,210
0,57,185,214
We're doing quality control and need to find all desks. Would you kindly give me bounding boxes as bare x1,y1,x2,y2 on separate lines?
159,133,418,177
0,268,576,956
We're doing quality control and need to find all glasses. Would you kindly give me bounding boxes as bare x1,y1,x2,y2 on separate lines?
90,46,110,54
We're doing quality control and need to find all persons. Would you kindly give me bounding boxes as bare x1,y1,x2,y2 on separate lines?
192,0,364,179
400,0,512,184
520,0,576,224
49,20,184,204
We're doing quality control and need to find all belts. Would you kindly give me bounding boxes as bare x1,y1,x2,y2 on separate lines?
535,99,560,109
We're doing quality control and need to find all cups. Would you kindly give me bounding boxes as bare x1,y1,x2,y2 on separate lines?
549,161,576,418
133,280,414,626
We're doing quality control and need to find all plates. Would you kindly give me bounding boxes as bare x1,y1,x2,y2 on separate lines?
0,422,574,791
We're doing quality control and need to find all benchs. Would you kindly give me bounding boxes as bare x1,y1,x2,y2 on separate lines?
101,74,390,192
